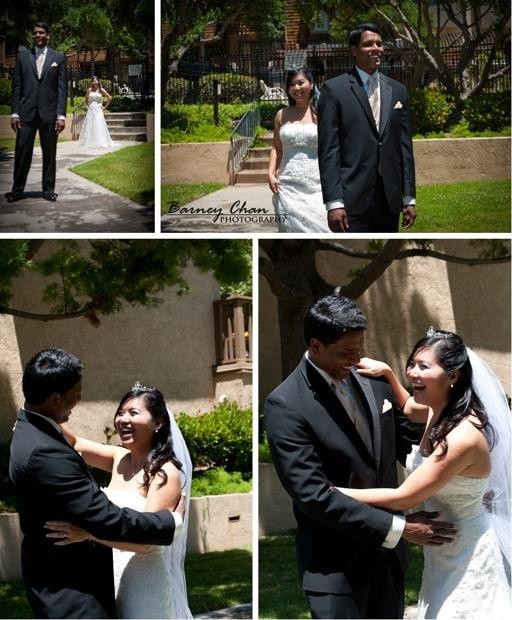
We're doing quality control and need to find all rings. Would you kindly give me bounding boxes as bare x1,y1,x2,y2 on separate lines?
63,534,68,538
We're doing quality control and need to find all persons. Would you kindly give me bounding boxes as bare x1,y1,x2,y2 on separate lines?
12,381,196,619
8,346,187,619
268,66,334,232
328,325,511,619
6,21,68,202
265,295,493,619
76,76,112,150
317,21,417,233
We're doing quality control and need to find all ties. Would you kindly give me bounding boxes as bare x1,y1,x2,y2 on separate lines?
366,77,379,131
36,49,44,78
